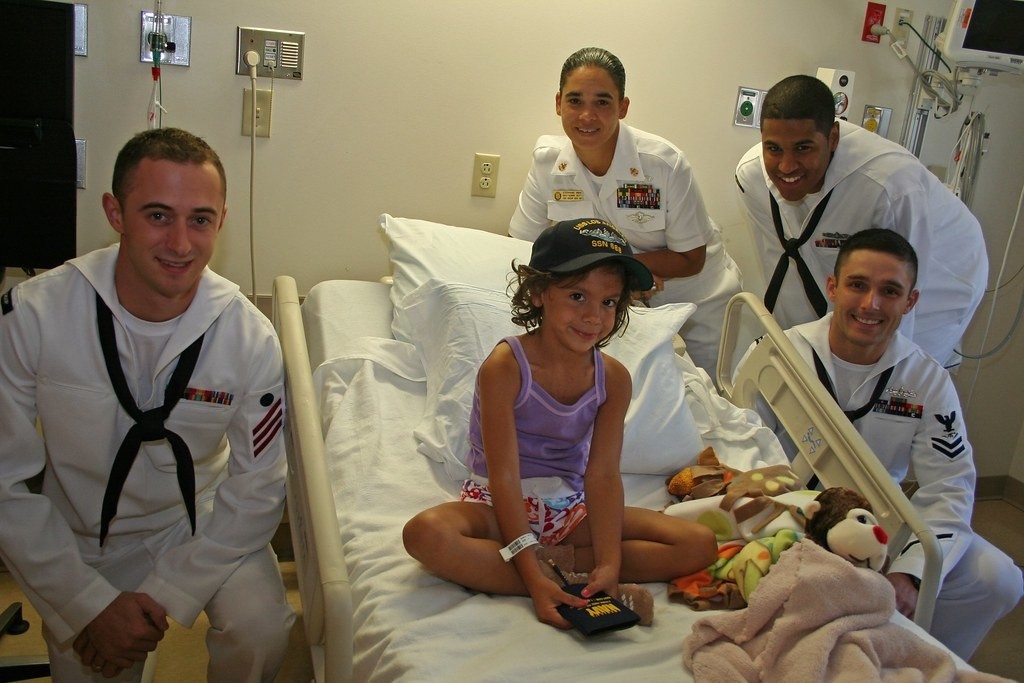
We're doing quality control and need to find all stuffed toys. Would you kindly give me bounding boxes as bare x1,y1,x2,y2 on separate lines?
662,487,890,573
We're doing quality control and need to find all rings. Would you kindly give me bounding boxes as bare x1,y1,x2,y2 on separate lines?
92,663,103,670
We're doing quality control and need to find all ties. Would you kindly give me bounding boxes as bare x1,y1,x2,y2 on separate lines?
763,187,834,319
94,290,206,548
812,346,895,423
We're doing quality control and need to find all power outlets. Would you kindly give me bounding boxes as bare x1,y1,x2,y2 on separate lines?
471,154,500,198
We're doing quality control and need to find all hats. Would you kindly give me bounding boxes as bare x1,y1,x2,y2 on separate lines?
529,218,654,293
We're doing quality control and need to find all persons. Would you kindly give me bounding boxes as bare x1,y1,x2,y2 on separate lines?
0,128,299,683
729,227,1024,662
734,75,989,370
401,218,717,625
508,47,742,381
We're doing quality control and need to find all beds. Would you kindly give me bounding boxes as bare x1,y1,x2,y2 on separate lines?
270,275,981,683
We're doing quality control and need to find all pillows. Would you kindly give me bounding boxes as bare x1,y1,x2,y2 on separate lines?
376,213,538,343
400,279,706,481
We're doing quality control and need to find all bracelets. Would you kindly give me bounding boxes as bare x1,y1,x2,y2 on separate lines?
499,533,542,564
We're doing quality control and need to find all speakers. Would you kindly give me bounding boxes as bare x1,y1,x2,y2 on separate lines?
0,0,78,270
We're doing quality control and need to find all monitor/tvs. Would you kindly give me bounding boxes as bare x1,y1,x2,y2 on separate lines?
943,0,1024,72
